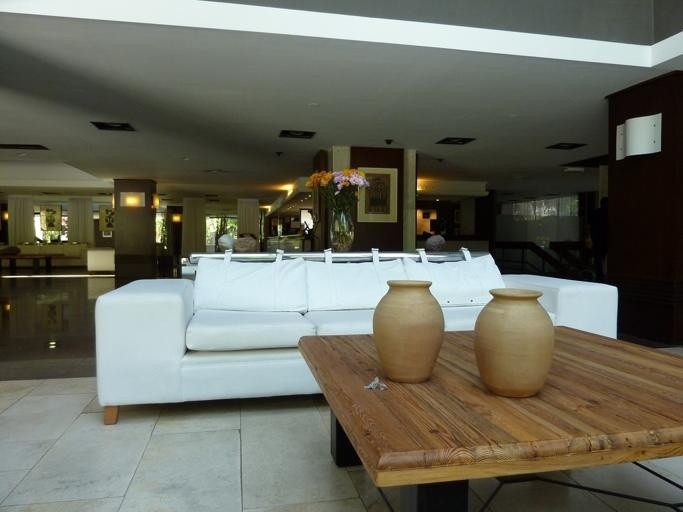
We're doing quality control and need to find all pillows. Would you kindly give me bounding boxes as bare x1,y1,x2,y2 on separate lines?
402,252,506,309
192,256,308,314
305,258,406,310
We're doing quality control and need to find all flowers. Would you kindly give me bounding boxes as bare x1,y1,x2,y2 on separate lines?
306,166,372,248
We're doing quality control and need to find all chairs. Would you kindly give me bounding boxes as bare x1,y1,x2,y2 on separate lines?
80,246,115,272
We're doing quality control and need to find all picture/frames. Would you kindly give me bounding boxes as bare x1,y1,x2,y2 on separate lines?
353,164,402,224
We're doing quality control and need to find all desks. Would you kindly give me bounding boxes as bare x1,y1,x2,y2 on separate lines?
1,253,63,275
294,319,682,511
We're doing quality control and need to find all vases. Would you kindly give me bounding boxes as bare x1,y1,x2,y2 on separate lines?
327,206,355,252
372,279,446,384
473,286,557,398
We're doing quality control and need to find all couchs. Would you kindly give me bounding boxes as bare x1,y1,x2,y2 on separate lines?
94,249,619,414
